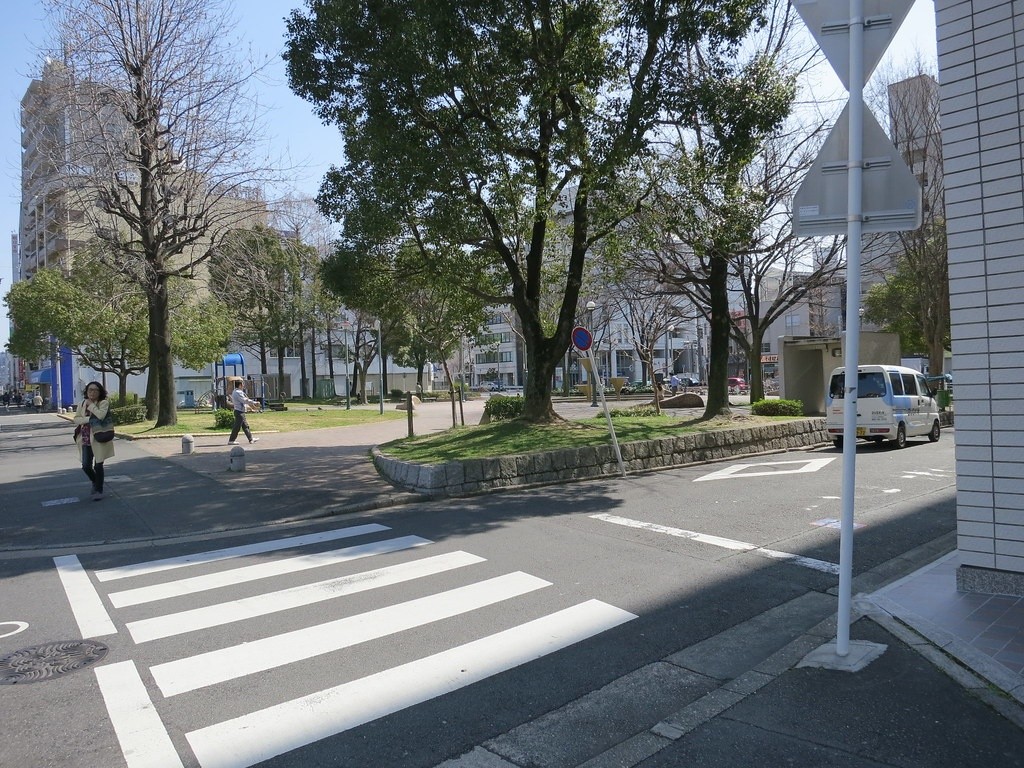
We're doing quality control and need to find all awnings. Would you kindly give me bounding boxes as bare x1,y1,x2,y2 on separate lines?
29,369,51,384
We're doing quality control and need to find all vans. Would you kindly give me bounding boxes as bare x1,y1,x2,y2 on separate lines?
826,365,941,448
680,377,699,387
728,378,746,390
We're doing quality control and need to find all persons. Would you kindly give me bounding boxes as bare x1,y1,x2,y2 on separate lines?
656,382,665,401
0,385,48,416
415,382,422,401
73,380,116,502
358,389,362,404
228,381,260,445
669,372,679,397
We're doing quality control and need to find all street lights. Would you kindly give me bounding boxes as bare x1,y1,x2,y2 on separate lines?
858,306,865,328
584,301,599,408
668,324,676,378
333,319,359,411
373,319,383,416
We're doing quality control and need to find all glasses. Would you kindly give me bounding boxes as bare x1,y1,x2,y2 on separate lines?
88,388,100,392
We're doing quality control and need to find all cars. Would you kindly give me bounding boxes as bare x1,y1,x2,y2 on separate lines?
481,382,504,391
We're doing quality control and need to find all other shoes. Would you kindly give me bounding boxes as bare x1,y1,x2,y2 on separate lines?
90,481,103,500
228,441,239,445
249,437,259,444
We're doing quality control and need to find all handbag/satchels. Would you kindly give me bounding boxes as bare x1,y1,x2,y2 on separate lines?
74,399,86,442
89,402,115,443
